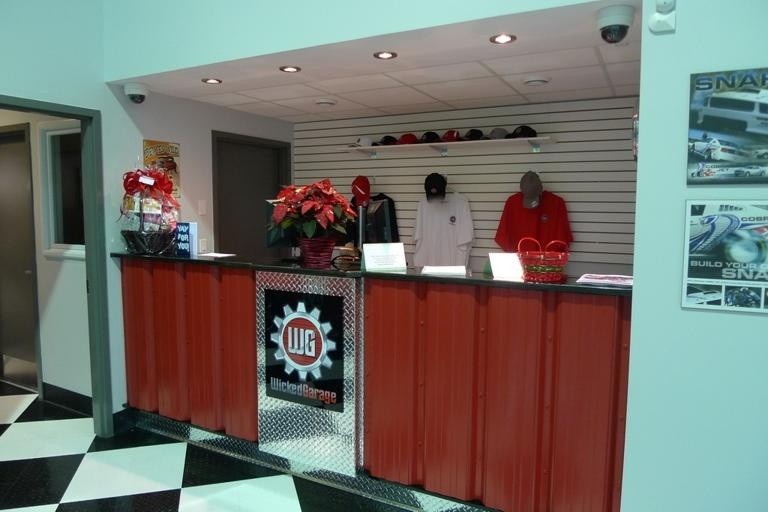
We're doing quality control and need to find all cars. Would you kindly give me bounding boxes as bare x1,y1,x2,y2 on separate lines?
736,166,766,177
753,148,768,159
689,138,751,164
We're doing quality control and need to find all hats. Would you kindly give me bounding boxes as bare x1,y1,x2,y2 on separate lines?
425,173,446,200
348,126,538,147
520,170,543,208
352,176,370,207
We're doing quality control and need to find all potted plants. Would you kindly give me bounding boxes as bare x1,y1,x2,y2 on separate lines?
264,176,358,269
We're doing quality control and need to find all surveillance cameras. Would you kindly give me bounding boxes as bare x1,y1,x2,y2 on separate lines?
596,7,636,44
125,85,149,104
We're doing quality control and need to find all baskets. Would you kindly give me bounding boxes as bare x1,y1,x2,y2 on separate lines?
518,237,568,282
302,238,336,269
